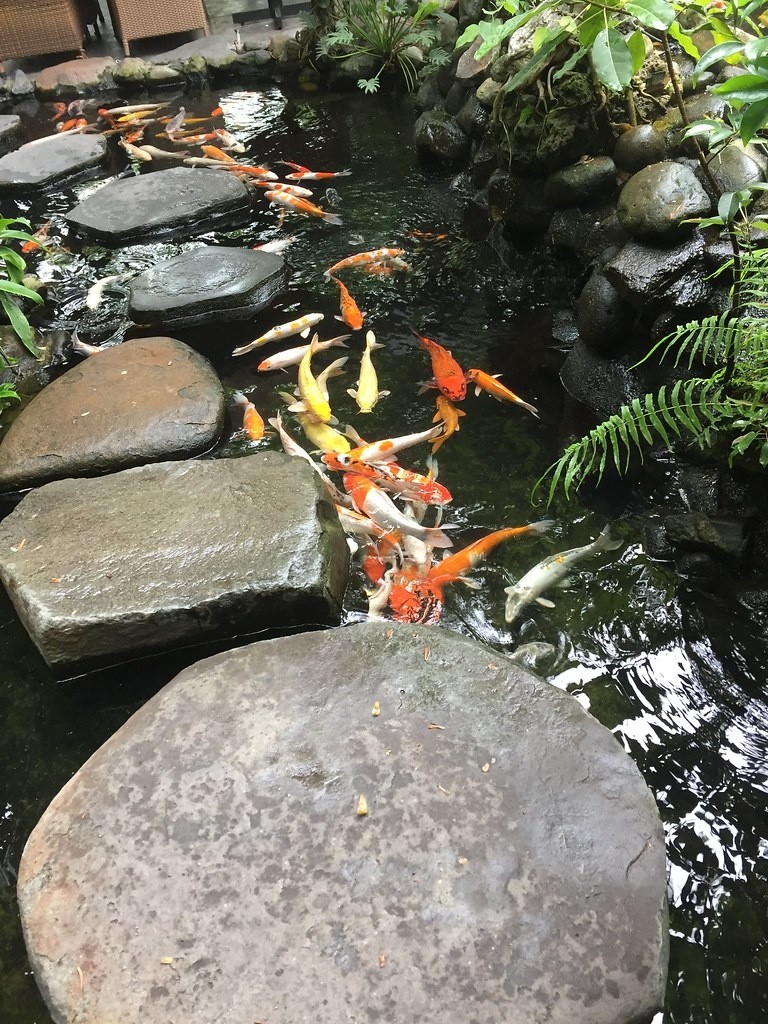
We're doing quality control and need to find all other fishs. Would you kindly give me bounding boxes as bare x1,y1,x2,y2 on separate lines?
20,98,624,669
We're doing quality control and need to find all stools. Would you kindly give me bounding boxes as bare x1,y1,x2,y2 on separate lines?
80,0,105,40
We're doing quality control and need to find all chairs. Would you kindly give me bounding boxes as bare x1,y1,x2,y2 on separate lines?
106,0,210,55
0,0,93,78
